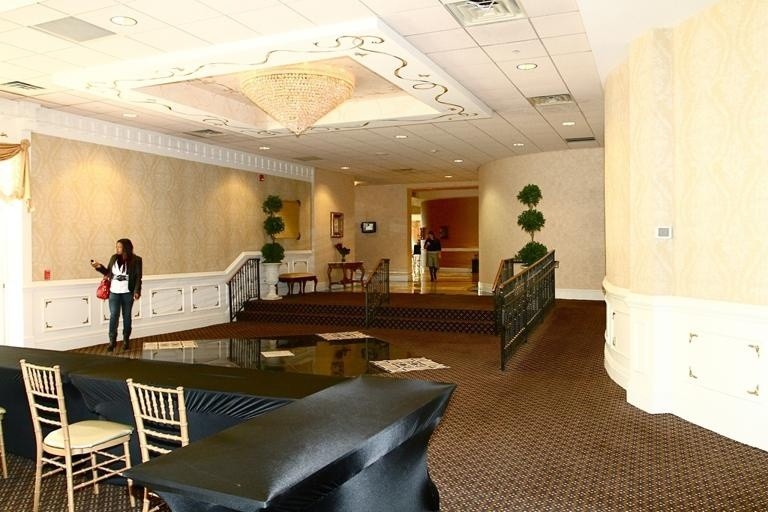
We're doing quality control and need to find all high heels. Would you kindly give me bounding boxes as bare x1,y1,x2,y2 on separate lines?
105,339,118,352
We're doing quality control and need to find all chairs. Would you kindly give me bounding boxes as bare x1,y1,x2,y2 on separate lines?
19,357,191,512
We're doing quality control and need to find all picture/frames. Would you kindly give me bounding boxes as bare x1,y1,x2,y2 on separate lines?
331,210,344,237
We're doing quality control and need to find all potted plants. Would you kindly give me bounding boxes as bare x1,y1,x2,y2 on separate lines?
261,195,283,301
514,183,548,310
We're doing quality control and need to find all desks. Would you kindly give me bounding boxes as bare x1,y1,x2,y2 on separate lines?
327,260,368,290
278,273,318,296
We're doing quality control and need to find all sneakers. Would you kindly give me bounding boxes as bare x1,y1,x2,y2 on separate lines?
123,340,129,350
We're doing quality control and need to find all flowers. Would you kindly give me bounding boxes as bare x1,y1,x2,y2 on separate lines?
336,244,352,262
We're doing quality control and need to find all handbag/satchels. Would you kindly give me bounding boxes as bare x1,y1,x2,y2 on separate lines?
95,277,112,299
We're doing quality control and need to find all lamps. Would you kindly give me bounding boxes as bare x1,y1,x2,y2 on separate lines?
236,65,355,139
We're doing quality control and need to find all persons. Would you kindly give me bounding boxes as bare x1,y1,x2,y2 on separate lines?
424,231,441,282
90,239,142,352
